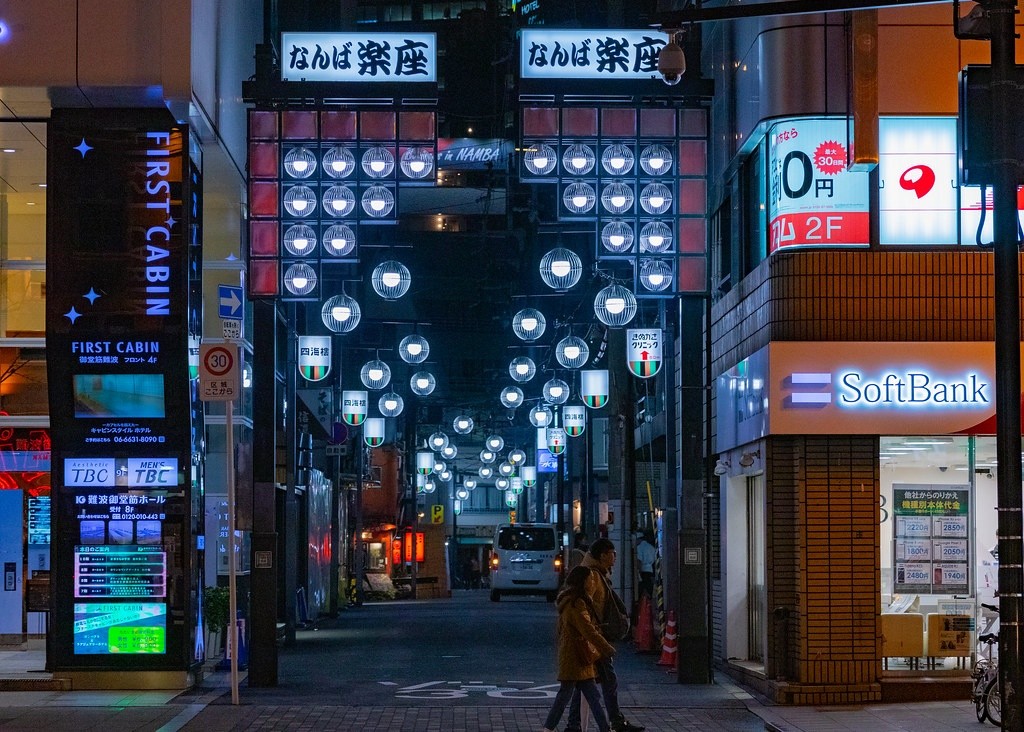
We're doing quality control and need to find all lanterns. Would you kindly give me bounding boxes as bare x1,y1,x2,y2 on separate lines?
392,539,401,564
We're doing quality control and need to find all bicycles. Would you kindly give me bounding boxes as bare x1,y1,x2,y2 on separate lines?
454,571,489,590
960,595,1006,726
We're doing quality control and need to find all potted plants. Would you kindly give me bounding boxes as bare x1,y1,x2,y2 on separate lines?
204,585,231,659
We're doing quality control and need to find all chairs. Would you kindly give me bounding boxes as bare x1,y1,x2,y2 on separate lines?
880,612,924,671
923,613,971,672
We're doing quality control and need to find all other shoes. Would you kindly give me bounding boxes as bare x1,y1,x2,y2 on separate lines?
610,721,646,732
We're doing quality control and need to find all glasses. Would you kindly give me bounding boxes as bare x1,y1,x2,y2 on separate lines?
607,550,617,557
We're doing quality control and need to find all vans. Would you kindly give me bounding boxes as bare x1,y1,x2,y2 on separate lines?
487,522,567,603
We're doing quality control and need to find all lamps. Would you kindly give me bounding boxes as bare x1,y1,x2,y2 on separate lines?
714,460,731,475
282,142,673,501
738,449,761,467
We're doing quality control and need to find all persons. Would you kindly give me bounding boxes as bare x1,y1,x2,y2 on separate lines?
463,556,479,590
565,538,645,732
544,565,617,732
572,533,588,565
638,532,656,630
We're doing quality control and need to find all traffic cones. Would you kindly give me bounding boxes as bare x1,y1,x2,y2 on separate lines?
632,592,653,651
656,607,677,666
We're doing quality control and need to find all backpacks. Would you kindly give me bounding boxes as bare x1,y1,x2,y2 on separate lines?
590,567,630,642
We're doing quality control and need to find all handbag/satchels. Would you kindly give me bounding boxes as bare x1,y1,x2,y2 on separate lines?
577,636,600,666
